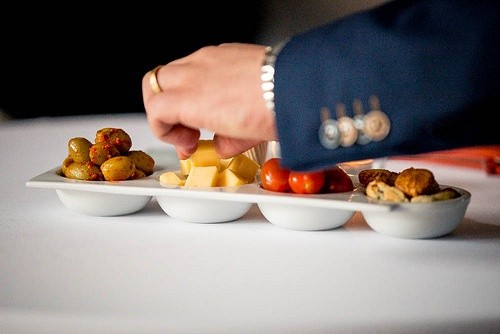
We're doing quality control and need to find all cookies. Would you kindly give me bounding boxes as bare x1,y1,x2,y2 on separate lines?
358,167,462,205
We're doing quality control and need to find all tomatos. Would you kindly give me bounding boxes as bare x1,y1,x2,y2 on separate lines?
260,158,353,195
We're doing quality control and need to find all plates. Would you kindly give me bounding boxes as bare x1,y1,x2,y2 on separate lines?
27,158,470,238
